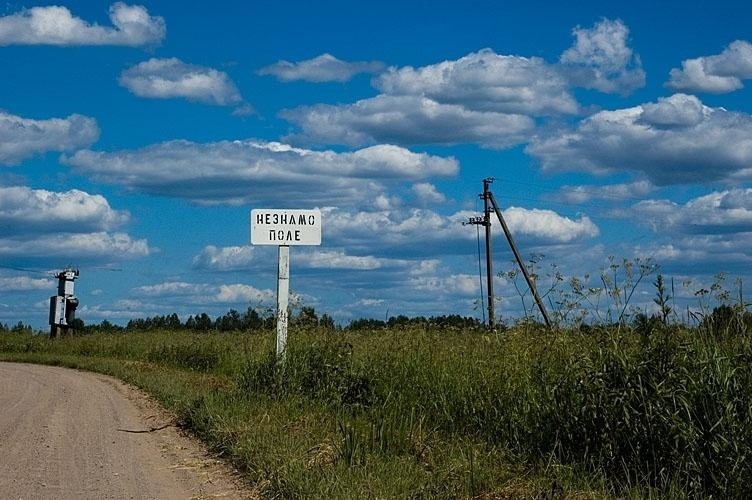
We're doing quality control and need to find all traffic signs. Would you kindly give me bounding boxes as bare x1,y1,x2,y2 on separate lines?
251,209,321,246
